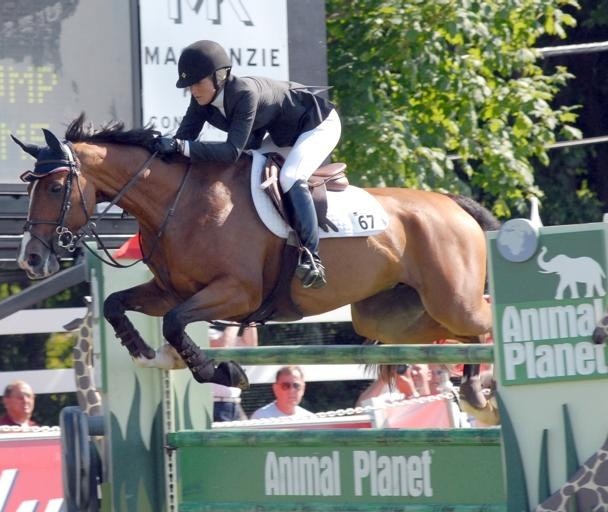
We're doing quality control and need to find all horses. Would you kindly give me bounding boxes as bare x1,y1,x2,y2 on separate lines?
10,109,495,412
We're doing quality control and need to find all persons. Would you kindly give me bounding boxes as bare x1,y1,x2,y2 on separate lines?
250,365,313,420
356,338,428,408
426,340,467,396
0,379,39,427
156,39,342,289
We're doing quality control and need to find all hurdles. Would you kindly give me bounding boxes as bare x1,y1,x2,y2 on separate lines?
59,219,608,512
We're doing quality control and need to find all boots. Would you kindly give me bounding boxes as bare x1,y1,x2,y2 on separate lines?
281,179,327,288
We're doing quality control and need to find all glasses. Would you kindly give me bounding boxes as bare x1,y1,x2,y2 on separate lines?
281,382,302,391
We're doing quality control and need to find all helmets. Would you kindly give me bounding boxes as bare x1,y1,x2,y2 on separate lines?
176,41,232,88
